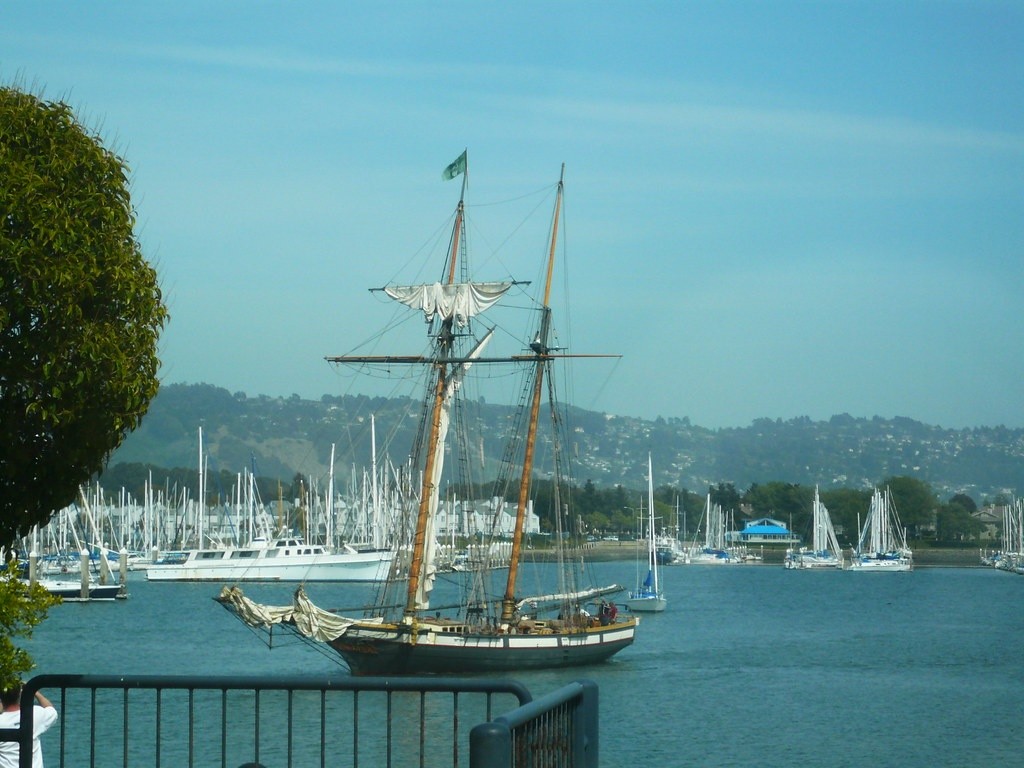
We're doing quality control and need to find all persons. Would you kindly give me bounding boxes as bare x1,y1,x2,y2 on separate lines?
598,599,617,626
0,679,58,768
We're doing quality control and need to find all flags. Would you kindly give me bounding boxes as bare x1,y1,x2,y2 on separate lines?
443,151,466,182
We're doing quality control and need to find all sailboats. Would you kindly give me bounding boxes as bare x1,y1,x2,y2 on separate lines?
646,483,916,573
0,412,538,603
622,447,668,612
212,144,643,673
981,494,1024,574
